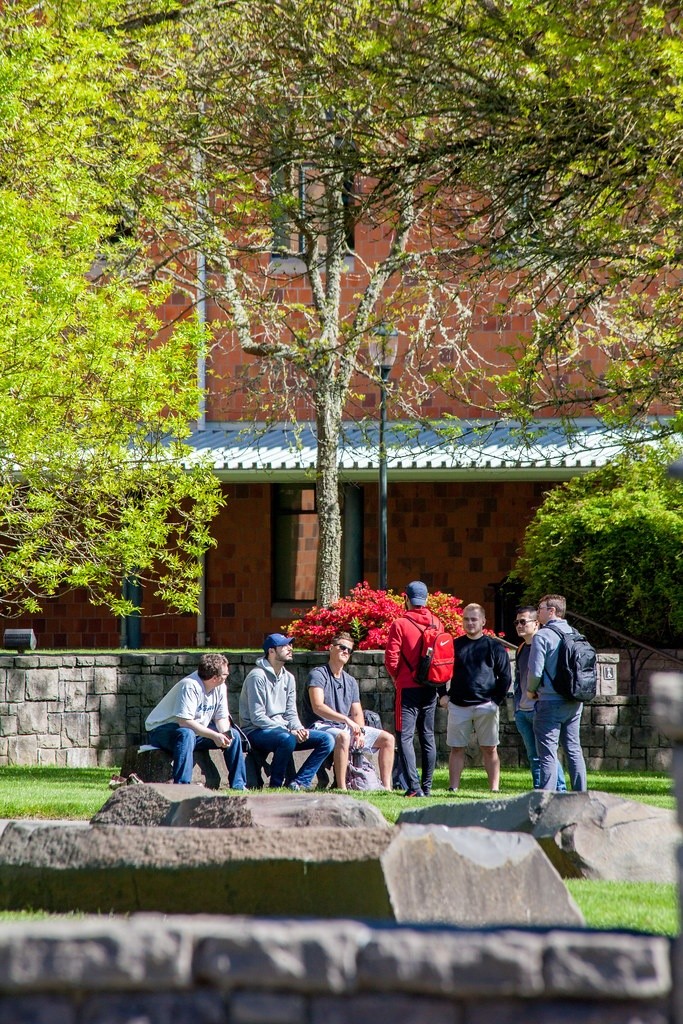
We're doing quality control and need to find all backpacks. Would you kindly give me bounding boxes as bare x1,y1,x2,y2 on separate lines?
363,709,382,731
346,754,385,791
542,624,597,702
400,615,455,688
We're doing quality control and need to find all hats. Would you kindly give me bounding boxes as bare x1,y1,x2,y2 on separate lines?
263,634,295,654
407,581,428,607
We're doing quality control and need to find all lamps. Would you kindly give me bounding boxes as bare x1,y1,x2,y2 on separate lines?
2,628,37,655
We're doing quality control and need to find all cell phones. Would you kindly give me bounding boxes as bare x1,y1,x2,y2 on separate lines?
222,730,233,740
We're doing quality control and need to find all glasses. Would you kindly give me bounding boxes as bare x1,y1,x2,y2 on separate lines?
212,671,230,677
277,643,293,650
333,644,354,654
514,619,538,626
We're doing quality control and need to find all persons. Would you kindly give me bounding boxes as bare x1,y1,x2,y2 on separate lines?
145,653,248,791
303,632,395,790
239,634,335,791
514,606,567,791
436,604,512,792
385,581,444,797
526,595,587,791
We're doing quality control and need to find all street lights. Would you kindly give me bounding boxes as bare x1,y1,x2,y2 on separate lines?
367,315,399,589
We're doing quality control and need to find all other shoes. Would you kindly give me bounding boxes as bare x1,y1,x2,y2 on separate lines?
288,781,304,792
403,788,430,797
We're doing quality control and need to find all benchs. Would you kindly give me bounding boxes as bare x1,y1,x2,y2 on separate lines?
124,746,399,793
